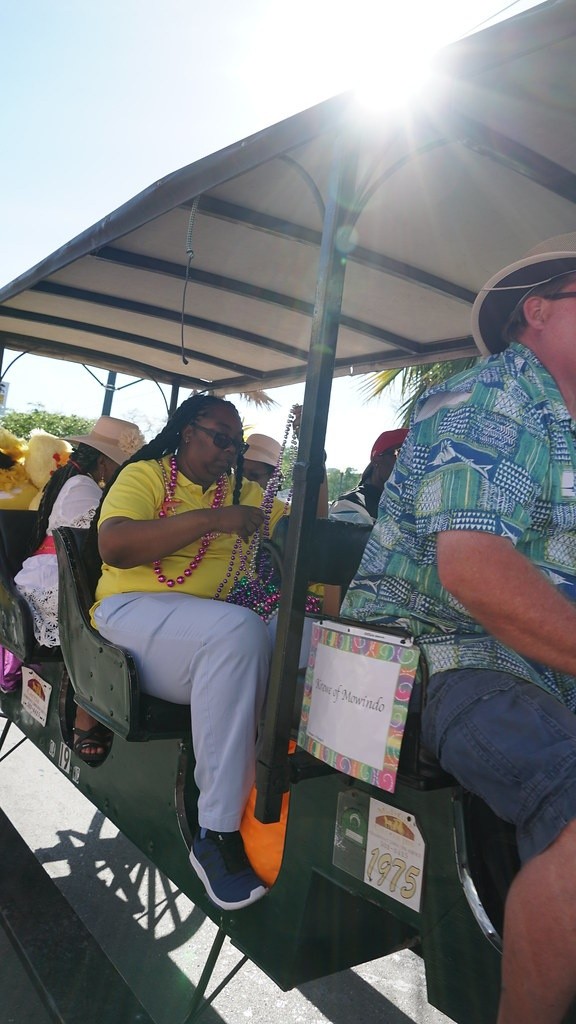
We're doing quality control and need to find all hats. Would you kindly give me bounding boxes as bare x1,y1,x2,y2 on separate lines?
370,428,409,463
232,434,285,483
468,233,576,356
55,415,145,466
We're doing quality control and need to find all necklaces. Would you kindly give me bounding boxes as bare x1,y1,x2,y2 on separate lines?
210,402,321,623
150,454,232,586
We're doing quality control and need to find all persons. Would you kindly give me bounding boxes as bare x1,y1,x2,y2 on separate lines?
236,431,294,507
86,393,329,912
337,251,576,1024
15,415,146,761
323,427,410,619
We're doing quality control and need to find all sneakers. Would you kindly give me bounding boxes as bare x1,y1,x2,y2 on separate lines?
188,827,269,910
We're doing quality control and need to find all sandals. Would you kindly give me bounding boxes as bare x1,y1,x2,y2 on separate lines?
72,719,107,761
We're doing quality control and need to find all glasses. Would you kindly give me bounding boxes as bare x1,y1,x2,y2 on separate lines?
378,447,403,459
188,420,250,456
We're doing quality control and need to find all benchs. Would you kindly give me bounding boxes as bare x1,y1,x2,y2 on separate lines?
52,526,191,741
0,508,63,662
272,516,519,929
